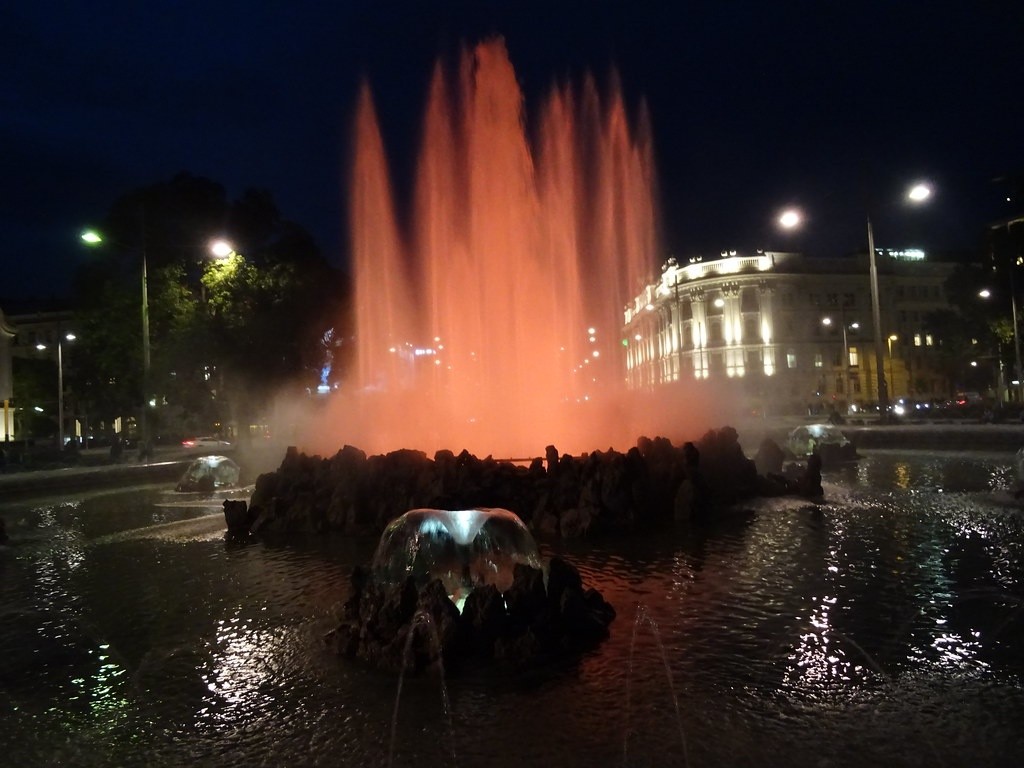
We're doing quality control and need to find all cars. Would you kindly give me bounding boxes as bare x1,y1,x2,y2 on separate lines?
845,390,982,420
181,436,230,451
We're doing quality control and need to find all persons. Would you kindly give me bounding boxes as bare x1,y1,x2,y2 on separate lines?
111,436,123,455
65,433,83,449
134,441,148,466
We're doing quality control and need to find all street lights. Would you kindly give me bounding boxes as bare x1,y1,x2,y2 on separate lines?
887,335,898,401
979,290,1022,403
779,185,930,423
36,333,76,451
822,318,860,400
81,230,232,464
